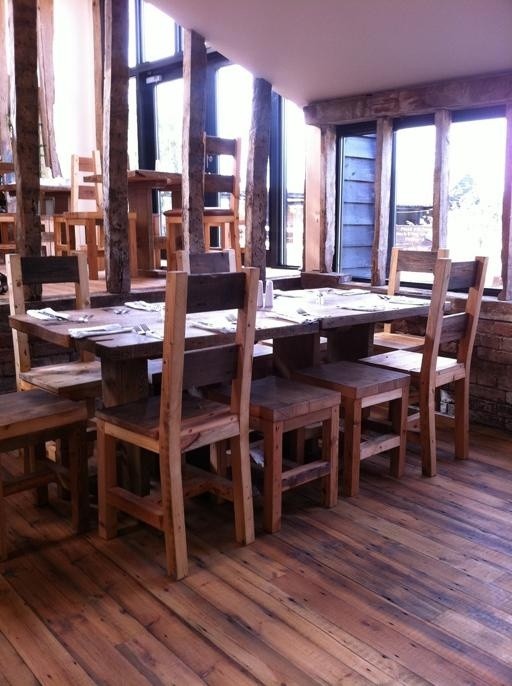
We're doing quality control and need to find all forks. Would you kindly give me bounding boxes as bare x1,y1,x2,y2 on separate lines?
225,313,238,324
54,313,94,324
133,323,164,339
